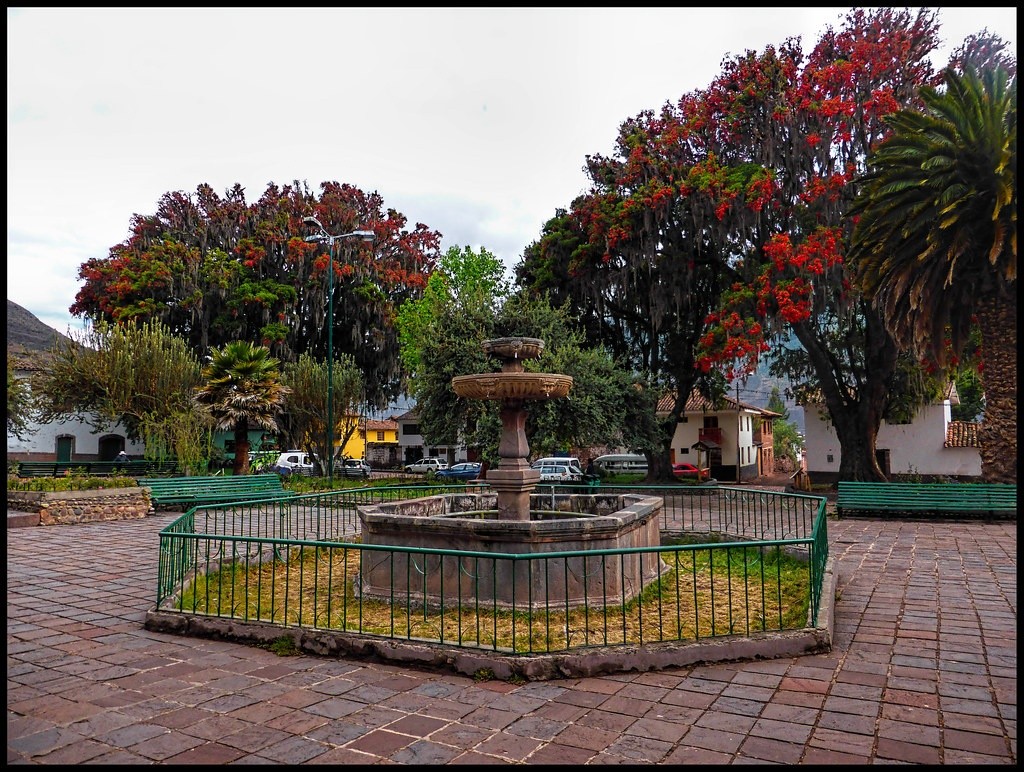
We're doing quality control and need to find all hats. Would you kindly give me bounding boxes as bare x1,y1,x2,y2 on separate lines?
119,451,127,456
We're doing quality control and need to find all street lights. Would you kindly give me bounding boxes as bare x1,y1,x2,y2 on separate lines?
300,216,375,486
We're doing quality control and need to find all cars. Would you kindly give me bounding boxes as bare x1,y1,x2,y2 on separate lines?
404,458,449,474
531,465,583,480
343,459,372,478
672,463,706,478
435,463,482,482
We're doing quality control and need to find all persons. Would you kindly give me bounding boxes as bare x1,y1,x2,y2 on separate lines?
113,451,130,462
585,458,594,474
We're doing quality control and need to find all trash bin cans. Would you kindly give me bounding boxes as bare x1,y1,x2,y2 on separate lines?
579,474,601,494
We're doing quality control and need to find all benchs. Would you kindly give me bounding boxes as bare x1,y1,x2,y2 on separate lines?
17,461,198,477
836,481,1017,520
136,474,296,512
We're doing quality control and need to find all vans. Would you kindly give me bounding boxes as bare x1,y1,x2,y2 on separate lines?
531,457,585,471
584,454,649,475
274,452,324,475
